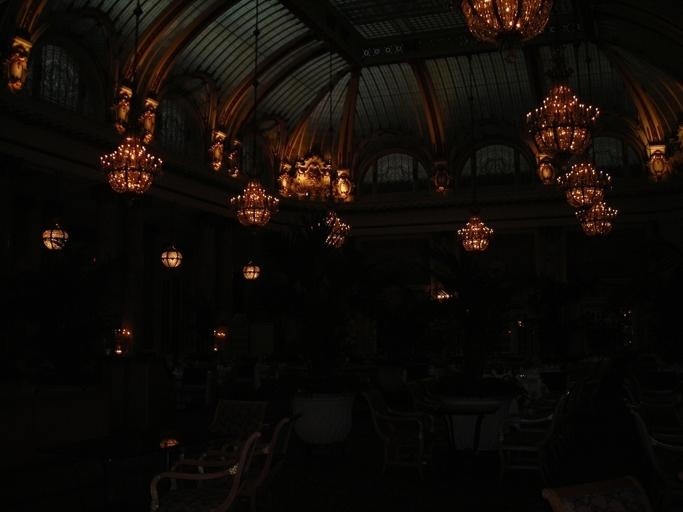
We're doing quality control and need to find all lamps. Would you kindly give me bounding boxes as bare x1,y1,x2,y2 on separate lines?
455,49,494,254
241,236,262,282
228,0,354,248
456,0,551,49
159,200,185,270
97,0,165,196
41,172,70,253
526,1,621,239
